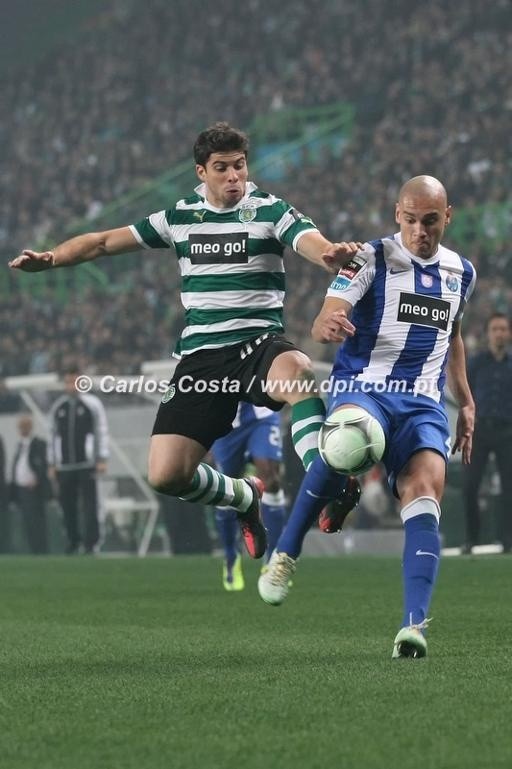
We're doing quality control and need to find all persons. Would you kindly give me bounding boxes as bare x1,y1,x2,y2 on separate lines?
44,365,109,553
5,119,364,559
255,171,477,664
7,413,53,555
202,400,298,595
2,0,512,414
454,313,511,555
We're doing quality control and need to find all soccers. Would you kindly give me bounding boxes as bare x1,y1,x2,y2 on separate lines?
318,408,385,477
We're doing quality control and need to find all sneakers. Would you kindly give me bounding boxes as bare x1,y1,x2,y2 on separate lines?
319,477,361,533
239,475,269,557
257,549,299,606
391,625,427,659
222,554,244,590
